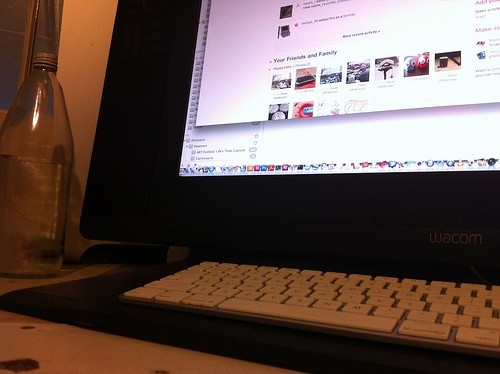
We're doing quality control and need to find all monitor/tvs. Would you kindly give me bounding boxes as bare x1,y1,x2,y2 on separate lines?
78,0,500,285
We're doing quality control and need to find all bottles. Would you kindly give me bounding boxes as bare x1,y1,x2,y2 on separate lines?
0,53,75,278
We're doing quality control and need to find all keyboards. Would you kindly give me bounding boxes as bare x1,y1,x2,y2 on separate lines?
117,260,500,358
